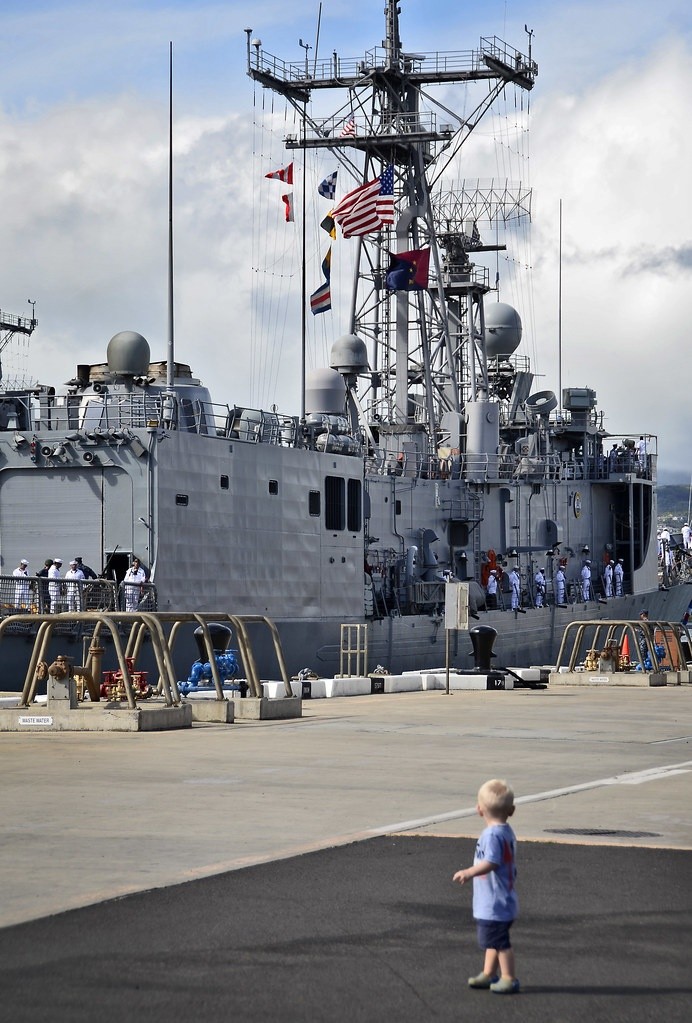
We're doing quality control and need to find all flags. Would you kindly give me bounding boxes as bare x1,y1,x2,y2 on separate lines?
264,161,293,184
310,280,331,316
282,192,294,222
332,158,395,239
322,246,332,284
318,170,338,200
340,111,358,137
320,207,336,240
384,247,430,291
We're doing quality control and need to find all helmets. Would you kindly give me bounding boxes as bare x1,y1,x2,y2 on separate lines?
45,559,53,565
641,609,649,616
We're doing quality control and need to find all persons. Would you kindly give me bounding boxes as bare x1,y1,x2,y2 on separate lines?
633,436,650,473
13,556,99,615
452,779,519,994
681,523,692,549
609,443,618,472
486,557,625,612
123,558,146,612
515,52,522,72
657,528,676,575
633,608,654,662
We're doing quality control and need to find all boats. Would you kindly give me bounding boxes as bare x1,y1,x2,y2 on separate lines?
0,0,692,694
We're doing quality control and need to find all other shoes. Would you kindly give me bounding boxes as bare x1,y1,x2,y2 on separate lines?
488,977,519,994
467,972,500,988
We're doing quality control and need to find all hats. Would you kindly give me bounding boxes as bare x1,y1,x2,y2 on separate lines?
684,523,688,525
70,561,78,565
134,558,139,563
540,567,545,570
514,565,519,569
560,566,565,568
663,528,668,530
610,560,614,563
21,559,29,565
490,570,497,574
55,558,64,563
619,558,624,561
75,557,83,562
586,560,591,564
